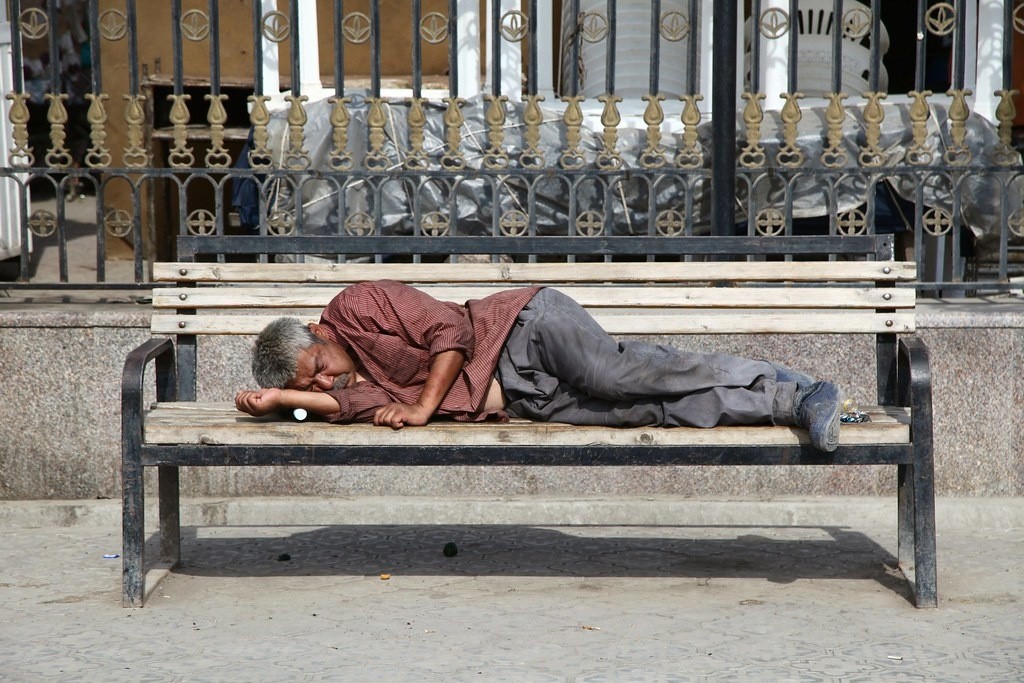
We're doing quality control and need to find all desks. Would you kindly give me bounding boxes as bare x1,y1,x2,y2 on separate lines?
140,55,527,284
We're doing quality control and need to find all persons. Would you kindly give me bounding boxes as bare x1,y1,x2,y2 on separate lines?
235,279,843,453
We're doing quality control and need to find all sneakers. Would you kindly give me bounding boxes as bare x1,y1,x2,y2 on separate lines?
801,381,840,452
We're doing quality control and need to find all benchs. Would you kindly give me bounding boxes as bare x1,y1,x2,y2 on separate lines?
117,235,939,616
